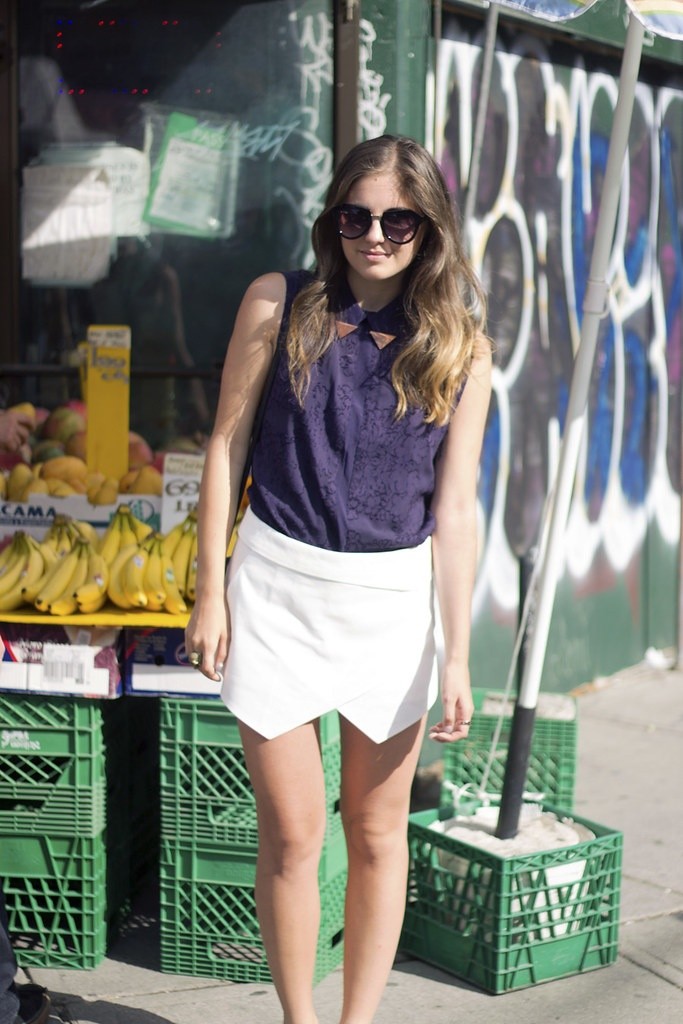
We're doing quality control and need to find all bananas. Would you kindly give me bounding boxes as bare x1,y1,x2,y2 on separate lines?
0,501,243,616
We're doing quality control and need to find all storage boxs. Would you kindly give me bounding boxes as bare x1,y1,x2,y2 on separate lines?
0,454,623,994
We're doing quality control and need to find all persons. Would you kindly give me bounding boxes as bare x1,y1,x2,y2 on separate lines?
184,138,491,1023
0,413,34,452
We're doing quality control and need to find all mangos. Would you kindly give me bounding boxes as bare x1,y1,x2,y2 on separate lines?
0,455,163,506
7,402,36,419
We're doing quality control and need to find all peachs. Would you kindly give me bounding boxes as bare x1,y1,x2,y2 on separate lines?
0,399,164,472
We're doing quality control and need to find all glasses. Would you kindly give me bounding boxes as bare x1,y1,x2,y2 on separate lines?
334,203,428,244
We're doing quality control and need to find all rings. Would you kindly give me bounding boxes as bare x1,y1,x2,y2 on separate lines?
459,721,470,724
190,652,200,668
